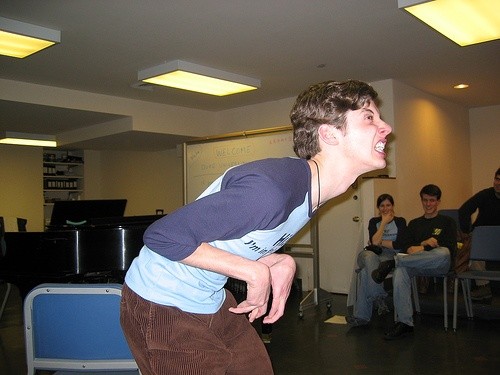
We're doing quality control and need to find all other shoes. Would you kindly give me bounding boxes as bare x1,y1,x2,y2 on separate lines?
396,324,414,336
472,286,493,300
371,260,394,284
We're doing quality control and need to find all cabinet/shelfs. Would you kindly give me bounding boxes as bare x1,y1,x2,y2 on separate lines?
42,149,83,227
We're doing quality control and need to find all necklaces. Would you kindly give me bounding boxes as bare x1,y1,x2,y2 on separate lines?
308,159,324,208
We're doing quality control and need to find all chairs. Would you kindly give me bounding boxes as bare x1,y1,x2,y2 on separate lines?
23,283,142,375
355,225,500,332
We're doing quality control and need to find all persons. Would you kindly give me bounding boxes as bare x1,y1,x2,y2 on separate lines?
371,184,457,340
458,168,500,304
345,193,406,327
120,78,392,375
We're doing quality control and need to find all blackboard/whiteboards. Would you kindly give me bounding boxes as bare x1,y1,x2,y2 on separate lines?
184,129,316,247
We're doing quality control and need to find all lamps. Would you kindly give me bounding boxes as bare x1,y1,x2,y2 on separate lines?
397,0,500,47
0,16,61,59
138,60,261,97
